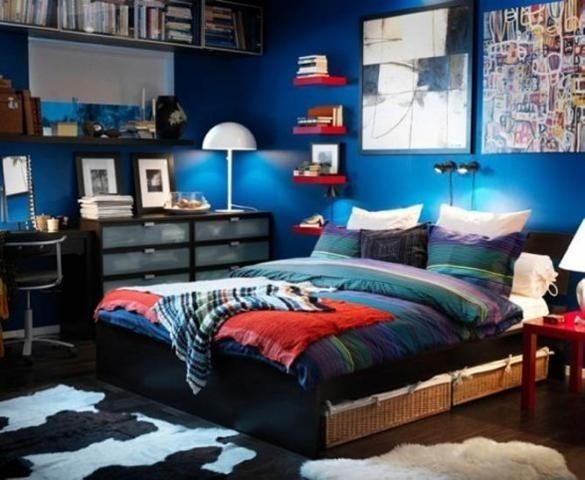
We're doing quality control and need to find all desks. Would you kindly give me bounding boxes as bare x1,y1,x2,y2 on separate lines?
2,231,94,340
522,310,584,412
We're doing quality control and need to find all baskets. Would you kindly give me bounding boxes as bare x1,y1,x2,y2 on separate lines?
451,347,555,405
323,372,452,447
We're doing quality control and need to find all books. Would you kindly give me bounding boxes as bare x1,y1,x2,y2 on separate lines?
290,53,329,77
295,102,345,129
291,161,321,177
297,213,325,228
0,0,250,54
75,192,139,219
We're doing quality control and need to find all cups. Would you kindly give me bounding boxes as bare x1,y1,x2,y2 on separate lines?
35,212,60,234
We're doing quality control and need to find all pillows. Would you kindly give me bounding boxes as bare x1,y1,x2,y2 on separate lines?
310,203,559,300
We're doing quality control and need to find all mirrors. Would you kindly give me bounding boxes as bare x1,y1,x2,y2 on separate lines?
3,155,29,197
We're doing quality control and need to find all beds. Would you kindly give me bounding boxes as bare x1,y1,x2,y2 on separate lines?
96,230,573,450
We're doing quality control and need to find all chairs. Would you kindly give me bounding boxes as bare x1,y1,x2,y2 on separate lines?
1,229,77,367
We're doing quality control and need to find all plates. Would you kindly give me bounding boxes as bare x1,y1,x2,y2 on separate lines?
163,207,212,215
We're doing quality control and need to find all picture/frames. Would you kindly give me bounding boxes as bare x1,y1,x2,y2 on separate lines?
74,152,176,215
360,0,473,155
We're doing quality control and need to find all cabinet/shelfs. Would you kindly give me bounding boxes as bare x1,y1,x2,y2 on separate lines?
294,77,347,235
1,0,264,57
77,212,273,337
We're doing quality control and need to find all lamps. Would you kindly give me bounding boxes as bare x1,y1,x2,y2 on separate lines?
558,218,585,310
435,161,479,209
202,122,257,212
